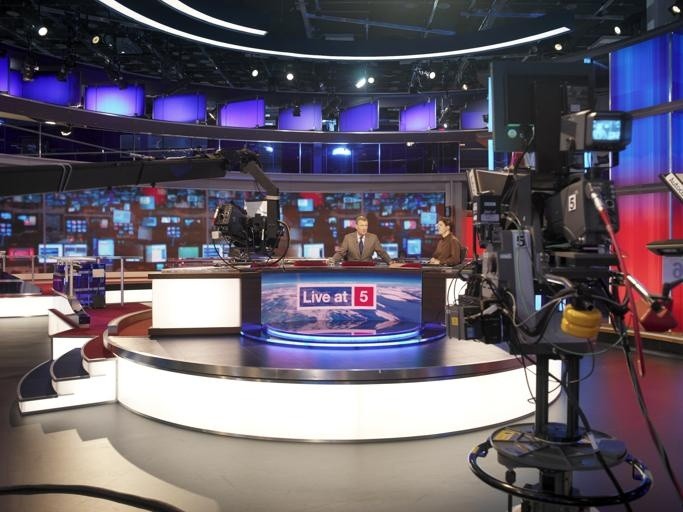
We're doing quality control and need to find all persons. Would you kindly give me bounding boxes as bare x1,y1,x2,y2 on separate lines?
326,216,399,266
427,218,461,267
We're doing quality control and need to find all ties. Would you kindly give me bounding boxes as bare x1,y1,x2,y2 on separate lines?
359,237,363,255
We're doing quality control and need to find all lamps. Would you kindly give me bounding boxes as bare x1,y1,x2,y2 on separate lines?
0,0,683,148
